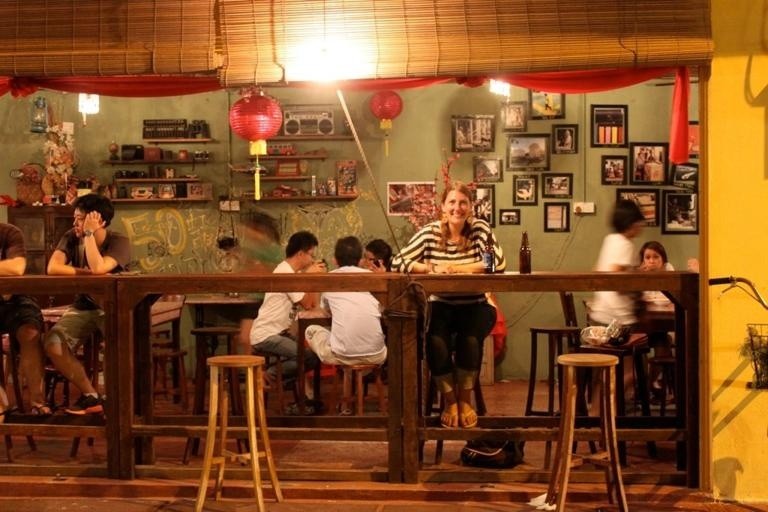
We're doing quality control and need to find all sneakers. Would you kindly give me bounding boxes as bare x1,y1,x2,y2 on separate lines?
337,403,352,415
286,404,313,414
66,395,102,414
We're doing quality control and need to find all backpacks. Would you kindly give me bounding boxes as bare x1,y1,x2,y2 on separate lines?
459,436,524,466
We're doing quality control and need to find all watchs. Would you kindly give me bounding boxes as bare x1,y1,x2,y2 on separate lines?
83,229,94,236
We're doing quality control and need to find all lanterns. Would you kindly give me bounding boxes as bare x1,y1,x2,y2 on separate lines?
228,88,283,200
370,89,403,157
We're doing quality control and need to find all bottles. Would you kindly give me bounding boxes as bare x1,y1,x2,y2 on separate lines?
519,230,532,273
483,232,496,274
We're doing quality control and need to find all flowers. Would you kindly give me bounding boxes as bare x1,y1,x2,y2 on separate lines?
39,125,75,178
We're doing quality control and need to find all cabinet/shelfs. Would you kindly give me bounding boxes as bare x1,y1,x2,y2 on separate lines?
5,204,76,332
101,136,216,204
249,132,357,204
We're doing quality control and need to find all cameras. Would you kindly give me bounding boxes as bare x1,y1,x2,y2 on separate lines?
217,236,238,251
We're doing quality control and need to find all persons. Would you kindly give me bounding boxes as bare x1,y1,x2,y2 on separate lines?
248,230,328,416
454,90,573,223
390,179,507,429
0,222,54,423
304,236,388,415
43,192,131,415
363,238,397,272
638,240,679,400
587,197,667,349
607,147,696,226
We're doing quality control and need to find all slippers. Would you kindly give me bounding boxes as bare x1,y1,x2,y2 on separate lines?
461,409,477,427
438,409,457,430
30,405,52,419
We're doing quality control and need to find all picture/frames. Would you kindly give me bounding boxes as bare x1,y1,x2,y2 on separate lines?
186,183,214,201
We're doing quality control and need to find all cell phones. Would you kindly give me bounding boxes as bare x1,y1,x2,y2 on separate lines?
374,258,381,268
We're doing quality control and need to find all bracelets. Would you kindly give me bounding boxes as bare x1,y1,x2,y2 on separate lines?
431,263,438,272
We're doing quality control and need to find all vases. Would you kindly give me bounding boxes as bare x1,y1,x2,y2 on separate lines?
41,175,67,197
